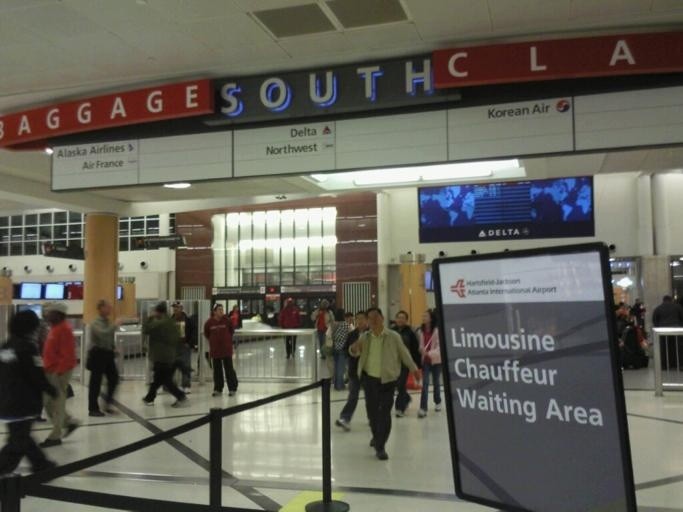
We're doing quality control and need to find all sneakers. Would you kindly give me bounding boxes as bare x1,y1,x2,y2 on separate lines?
435,401,442,411
375,445,388,459
34,421,79,469
212,390,236,397
89,410,104,416
396,410,403,416
142,385,191,409
417,409,426,417
335,418,350,429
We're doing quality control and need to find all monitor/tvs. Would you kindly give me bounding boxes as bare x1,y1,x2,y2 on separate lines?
20,282,43,299
44,283,65,300
117,285,123,300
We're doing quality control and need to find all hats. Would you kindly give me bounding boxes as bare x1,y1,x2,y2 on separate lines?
44,303,67,315
170,301,183,307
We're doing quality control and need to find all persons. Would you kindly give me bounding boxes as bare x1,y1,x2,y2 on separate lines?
279,295,301,359
336,309,371,433
85,297,120,416
348,305,422,459
263,305,277,326
615,295,682,372
141,298,197,409
229,303,243,329
0,298,81,482
203,303,239,398
310,295,443,417
